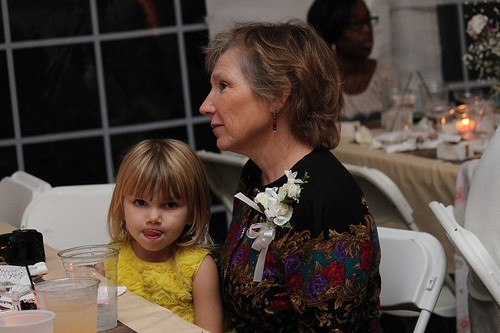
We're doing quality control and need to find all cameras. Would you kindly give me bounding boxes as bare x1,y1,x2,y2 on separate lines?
0,229,46,266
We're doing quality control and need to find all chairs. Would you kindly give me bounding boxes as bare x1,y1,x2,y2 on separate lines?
0,148,500,333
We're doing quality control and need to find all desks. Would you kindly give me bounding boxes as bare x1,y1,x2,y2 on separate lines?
22,183,118,252
220,123,460,274
0,221,213,333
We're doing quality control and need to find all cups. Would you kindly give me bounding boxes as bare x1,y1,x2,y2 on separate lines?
57,244,122,332
35,277,101,333
1,309,56,333
381,82,496,143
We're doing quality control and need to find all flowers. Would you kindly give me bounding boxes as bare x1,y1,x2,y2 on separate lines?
463,0,500,79
232,168,309,282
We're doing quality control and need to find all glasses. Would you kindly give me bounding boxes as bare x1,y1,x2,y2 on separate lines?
342,16,378,32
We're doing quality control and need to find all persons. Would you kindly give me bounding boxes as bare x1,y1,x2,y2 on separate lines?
198,18,381,333
95,139,222,333
307,0,400,128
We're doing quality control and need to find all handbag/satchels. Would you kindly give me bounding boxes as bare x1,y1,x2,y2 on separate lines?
0,229,46,266
0,262,48,309
